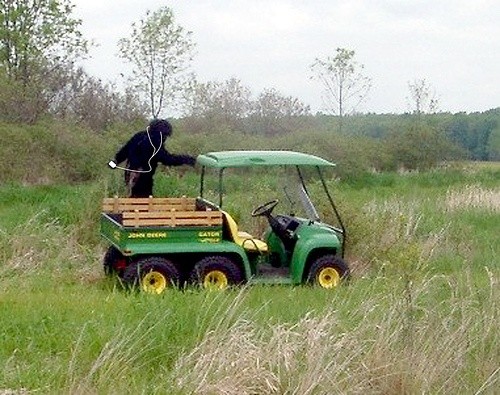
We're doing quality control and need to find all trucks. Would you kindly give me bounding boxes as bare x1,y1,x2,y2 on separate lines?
97,150,351,298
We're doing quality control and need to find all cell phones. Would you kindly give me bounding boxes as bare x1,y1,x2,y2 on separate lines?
105,159,117,170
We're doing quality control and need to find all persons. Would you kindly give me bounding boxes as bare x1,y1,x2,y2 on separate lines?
108,120,197,212
